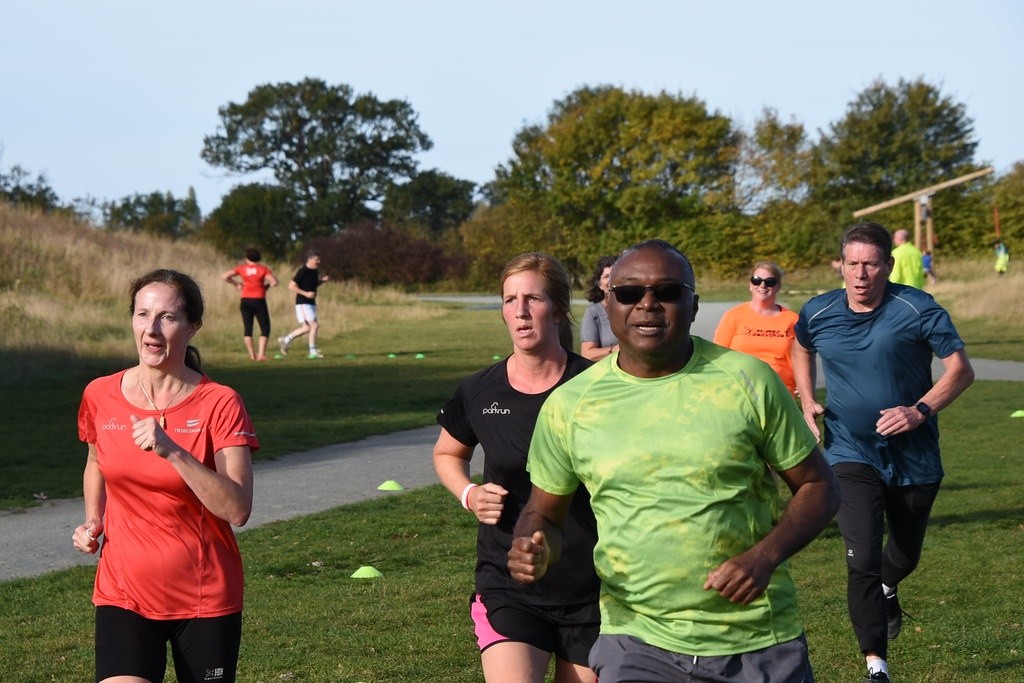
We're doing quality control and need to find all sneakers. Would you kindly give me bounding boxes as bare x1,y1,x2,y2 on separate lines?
880,583,915,640
862,665,891,683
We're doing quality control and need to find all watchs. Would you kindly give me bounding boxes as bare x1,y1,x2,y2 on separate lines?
914,401,938,418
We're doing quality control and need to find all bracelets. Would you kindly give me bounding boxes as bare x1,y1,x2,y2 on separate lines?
461,483,479,511
609,343,613,353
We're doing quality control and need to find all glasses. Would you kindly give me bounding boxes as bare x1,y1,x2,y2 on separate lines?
607,280,694,304
749,275,780,287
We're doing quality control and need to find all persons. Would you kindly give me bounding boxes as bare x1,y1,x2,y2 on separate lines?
72,270,261,683
888,229,925,291
432,252,602,683
831,258,847,288
790,225,975,683
579,258,622,365
712,261,800,405
277,248,330,360
993,239,1010,276
922,250,937,285
508,239,840,683
223,250,279,363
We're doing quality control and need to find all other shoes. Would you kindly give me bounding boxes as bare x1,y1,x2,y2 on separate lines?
278,337,290,355
308,353,325,359
248,354,270,361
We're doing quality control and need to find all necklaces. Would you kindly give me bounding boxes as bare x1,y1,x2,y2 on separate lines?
138,365,188,430
